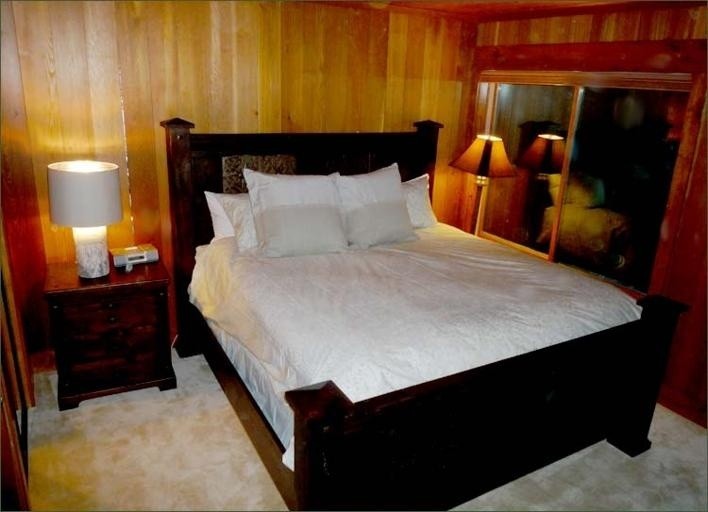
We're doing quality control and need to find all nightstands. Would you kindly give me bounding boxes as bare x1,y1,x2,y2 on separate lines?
39,262,178,412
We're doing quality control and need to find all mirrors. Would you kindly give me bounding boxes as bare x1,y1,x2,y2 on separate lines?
479,82,691,294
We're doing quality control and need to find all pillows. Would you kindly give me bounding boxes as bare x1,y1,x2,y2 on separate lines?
203,163,438,260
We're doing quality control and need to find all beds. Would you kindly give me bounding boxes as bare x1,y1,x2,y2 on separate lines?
158,117,689,510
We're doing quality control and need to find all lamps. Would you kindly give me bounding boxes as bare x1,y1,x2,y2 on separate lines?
446,133,517,235
514,133,565,246
46,159,124,279
